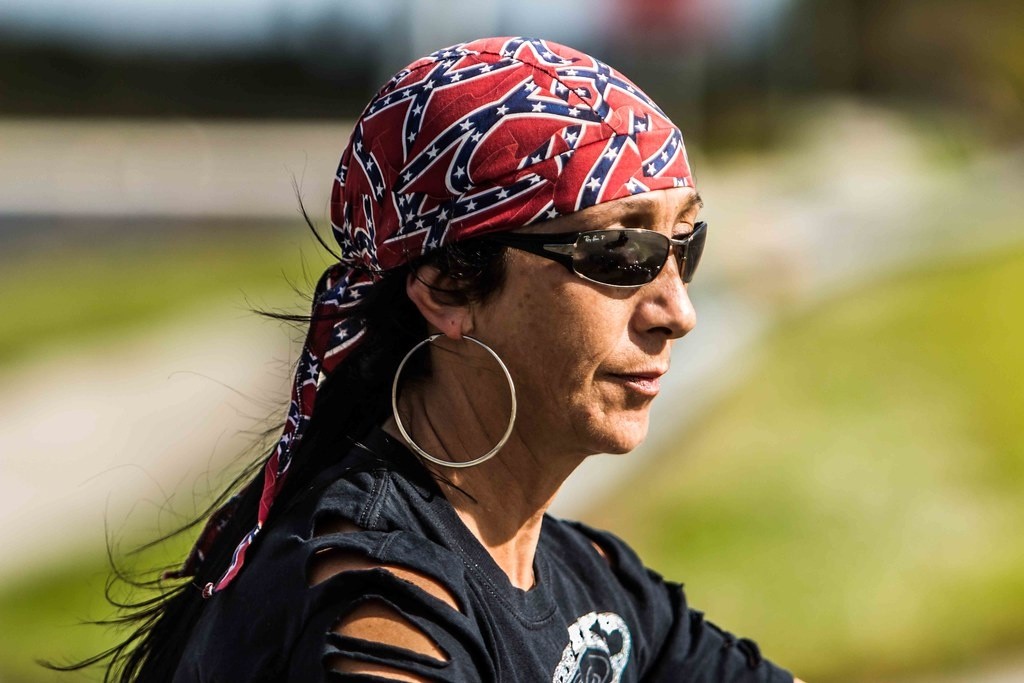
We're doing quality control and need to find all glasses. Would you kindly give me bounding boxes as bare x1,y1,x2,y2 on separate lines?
469,220,708,289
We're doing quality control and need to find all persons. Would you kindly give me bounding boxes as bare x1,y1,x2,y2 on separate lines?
121,37,806,682
